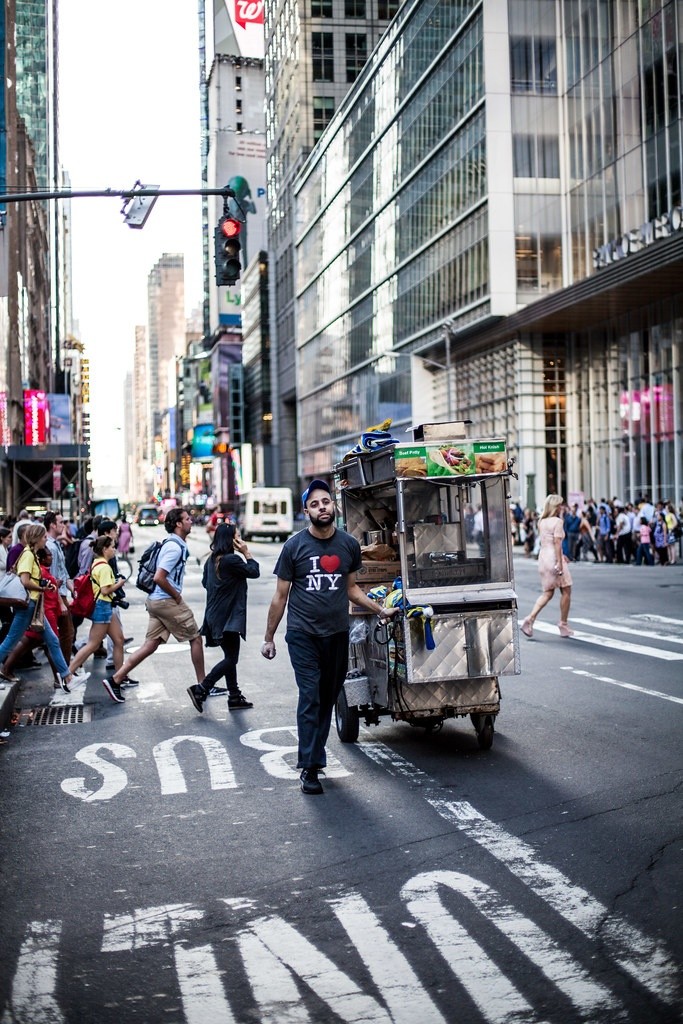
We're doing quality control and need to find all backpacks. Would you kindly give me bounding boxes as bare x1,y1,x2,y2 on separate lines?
137,539,188,593
69,562,108,617
65,537,93,579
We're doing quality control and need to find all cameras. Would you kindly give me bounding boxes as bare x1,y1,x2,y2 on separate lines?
111,596,129,609
39,580,50,586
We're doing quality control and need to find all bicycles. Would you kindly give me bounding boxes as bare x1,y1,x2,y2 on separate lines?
114,557,132,585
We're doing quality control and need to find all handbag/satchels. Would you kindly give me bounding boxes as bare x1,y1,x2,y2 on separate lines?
674,526,682,538
130,547,134,553
0,550,30,609
31,587,45,631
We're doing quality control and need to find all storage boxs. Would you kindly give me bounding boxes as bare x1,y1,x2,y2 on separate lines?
349,560,401,615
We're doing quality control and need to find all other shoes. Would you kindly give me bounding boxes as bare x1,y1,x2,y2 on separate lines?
0,636,134,687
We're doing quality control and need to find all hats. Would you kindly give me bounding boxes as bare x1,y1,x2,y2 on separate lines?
302,479,331,509
19,509,28,518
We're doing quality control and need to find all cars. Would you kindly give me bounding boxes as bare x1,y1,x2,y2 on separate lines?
135,506,178,526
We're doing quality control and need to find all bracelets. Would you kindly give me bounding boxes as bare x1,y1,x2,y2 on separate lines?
41,586,44,591
378,607,386,616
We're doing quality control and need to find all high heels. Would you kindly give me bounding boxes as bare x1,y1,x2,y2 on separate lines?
520,614,534,637
558,620,573,636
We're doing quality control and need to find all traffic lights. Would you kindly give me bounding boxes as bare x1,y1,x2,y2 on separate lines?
215,214,242,287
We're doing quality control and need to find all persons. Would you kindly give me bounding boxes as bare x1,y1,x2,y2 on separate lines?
261,480,400,796
0,509,139,693
457,496,683,566
186,523,260,713
520,495,573,637
102,508,227,703
172,504,246,565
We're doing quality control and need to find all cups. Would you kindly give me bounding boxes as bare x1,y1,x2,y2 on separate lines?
367,530,383,545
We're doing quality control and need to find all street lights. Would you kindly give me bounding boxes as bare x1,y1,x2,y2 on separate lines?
382,325,451,420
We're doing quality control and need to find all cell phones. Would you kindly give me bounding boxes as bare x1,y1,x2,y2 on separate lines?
233,540,240,548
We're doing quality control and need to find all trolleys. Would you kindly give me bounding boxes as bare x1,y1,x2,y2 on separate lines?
335,436,521,747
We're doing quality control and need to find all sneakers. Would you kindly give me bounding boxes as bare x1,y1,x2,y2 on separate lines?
0,684,5,690
208,684,228,695
67,672,91,690
103,676,125,703
228,693,253,710
300,768,325,794
119,676,138,687
187,683,209,713
56,672,70,694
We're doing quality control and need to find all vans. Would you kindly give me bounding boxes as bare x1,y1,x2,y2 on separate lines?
236,487,294,542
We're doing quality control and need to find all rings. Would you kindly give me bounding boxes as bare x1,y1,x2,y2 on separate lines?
555,566,562,575
